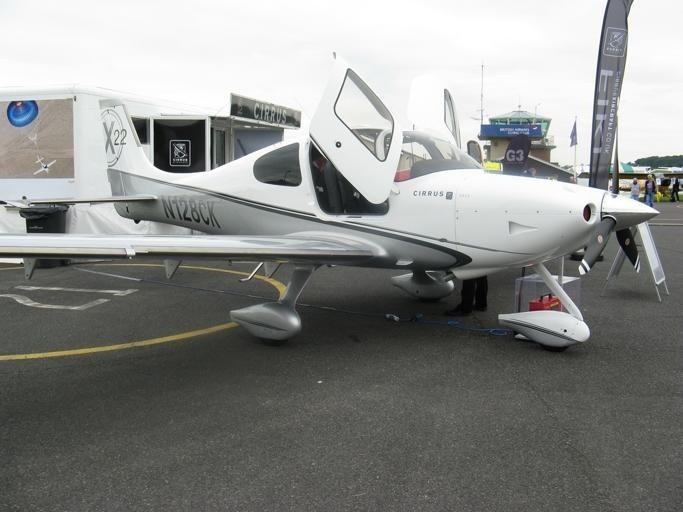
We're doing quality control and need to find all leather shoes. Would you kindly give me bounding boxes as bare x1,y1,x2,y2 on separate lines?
444,303,473,317
473,305,487,312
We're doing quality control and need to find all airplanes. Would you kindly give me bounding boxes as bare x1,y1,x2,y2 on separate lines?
0,51,661,352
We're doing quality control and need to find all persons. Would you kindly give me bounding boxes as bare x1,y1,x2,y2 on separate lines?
671,177,679,202
645,174,656,208
629,177,640,201
